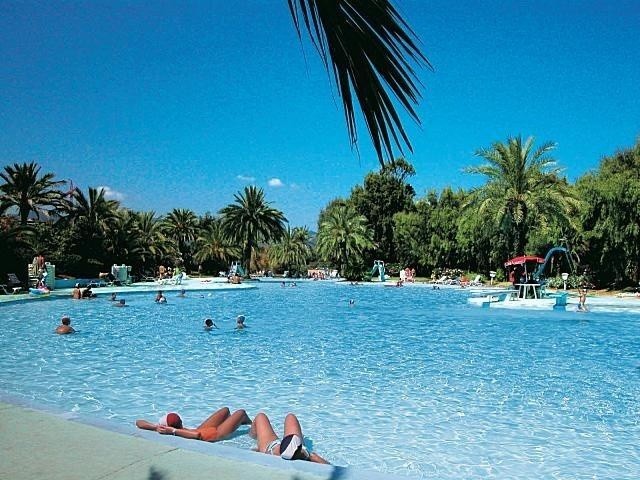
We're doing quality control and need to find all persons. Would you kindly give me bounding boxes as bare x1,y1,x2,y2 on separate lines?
385,263,540,298
140,265,145,274
71,283,94,299
199,265,328,288
99,272,122,286
42,287,50,294
578,287,591,312
57,318,75,335
33,253,45,288
154,290,167,304
176,289,185,297
158,264,186,280
205,315,248,329
134,407,253,441
109,292,129,307
248,412,330,465
349,298,354,304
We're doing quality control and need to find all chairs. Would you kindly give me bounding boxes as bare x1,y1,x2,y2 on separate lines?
429,275,484,287
138,272,181,286
1,271,29,295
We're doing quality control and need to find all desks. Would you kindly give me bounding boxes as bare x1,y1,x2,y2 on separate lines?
516,283,541,300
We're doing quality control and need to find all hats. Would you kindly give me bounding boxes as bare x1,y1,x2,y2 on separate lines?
161,413,181,427
280,434,302,460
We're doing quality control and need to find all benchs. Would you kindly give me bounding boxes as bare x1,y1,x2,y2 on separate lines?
87,282,114,288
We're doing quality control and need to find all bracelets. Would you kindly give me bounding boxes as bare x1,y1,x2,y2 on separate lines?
174,429,176,436
